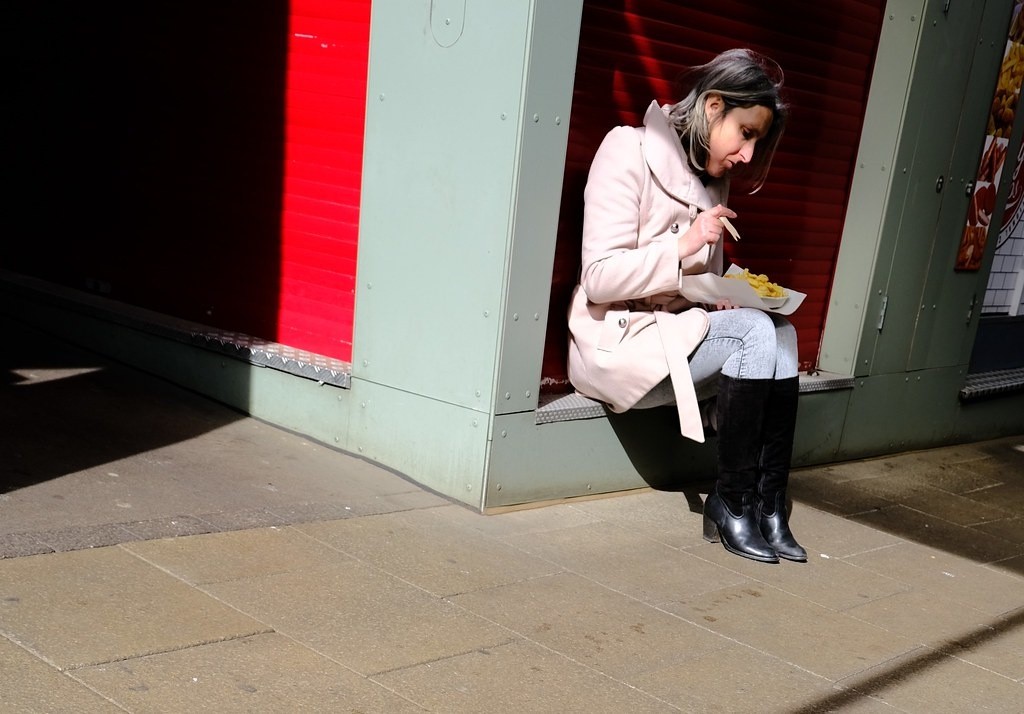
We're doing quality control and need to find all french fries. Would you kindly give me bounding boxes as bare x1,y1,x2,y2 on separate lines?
994,41,1024,93
719,269,785,299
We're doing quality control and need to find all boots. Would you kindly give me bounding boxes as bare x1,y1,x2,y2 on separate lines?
755,374,808,561
703,368,780,562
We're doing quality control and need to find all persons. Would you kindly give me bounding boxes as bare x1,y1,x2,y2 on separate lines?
566,49,806,563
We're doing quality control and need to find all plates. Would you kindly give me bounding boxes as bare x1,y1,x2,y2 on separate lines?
761,296,788,308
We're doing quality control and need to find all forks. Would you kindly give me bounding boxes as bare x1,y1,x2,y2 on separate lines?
719,217,741,242
